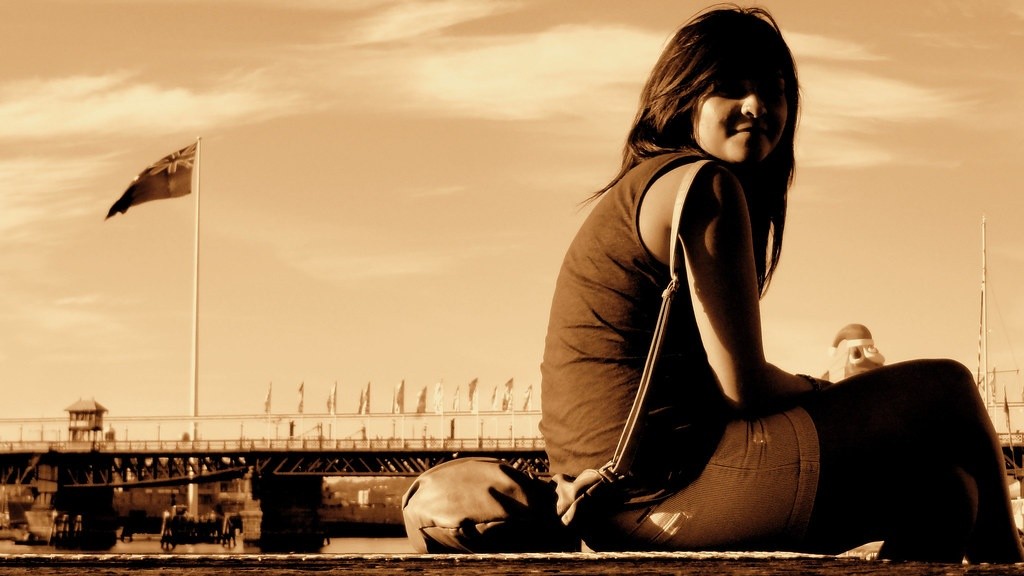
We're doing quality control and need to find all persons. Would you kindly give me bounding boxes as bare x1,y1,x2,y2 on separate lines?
536,7,1024,575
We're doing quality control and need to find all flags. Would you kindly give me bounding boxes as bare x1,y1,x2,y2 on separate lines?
104,141,198,218
261,379,537,416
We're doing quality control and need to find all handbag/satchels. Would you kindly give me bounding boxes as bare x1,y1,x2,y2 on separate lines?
400,457,631,554
